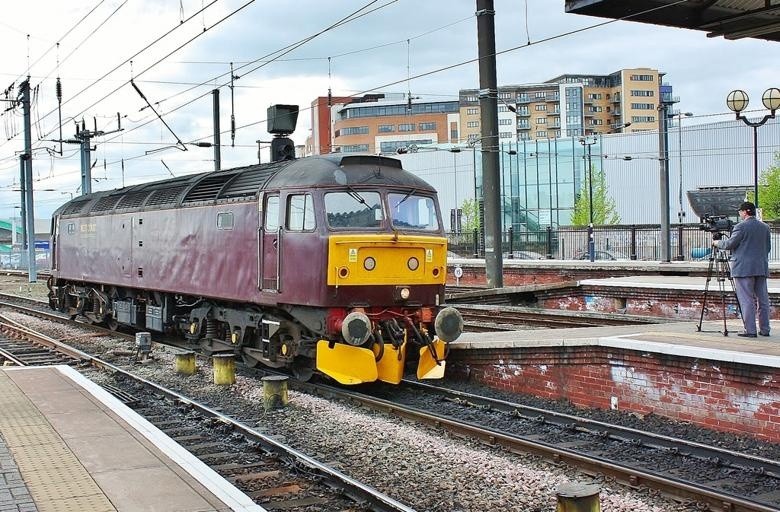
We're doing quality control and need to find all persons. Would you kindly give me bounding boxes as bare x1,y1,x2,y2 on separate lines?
711,202,771,337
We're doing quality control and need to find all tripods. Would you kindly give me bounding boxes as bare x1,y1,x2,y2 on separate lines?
695,244,746,337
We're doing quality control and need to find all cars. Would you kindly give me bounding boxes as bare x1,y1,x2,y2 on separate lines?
445,239,638,265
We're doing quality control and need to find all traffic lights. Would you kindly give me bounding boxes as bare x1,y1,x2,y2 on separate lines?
262,103,302,136
267,138,295,163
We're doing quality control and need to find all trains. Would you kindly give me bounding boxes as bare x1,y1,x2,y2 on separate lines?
46,153,465,386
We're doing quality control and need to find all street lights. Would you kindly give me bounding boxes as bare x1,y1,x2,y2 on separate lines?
722,83,779,234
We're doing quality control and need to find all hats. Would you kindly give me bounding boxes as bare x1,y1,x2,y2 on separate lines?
735,200,755,211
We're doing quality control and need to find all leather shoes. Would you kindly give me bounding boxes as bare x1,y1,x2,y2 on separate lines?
759,331,770,337
737,332,757,338
